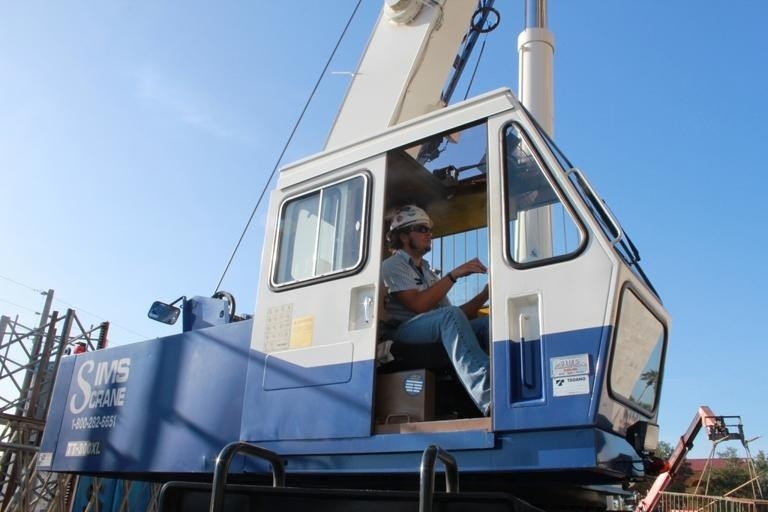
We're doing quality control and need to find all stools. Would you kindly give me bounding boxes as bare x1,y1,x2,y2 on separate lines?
377,342,438,422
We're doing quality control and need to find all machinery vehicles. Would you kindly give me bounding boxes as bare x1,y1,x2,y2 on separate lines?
34,1,671,510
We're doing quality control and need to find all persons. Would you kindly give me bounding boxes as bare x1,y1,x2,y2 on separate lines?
378,203,493,420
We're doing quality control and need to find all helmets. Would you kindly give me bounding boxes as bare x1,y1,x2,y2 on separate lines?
390,205,433,231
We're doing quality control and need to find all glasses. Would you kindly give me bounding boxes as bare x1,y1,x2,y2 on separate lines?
403,224,433,235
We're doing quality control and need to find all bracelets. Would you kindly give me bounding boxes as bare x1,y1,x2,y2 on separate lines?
447,271,457,284
